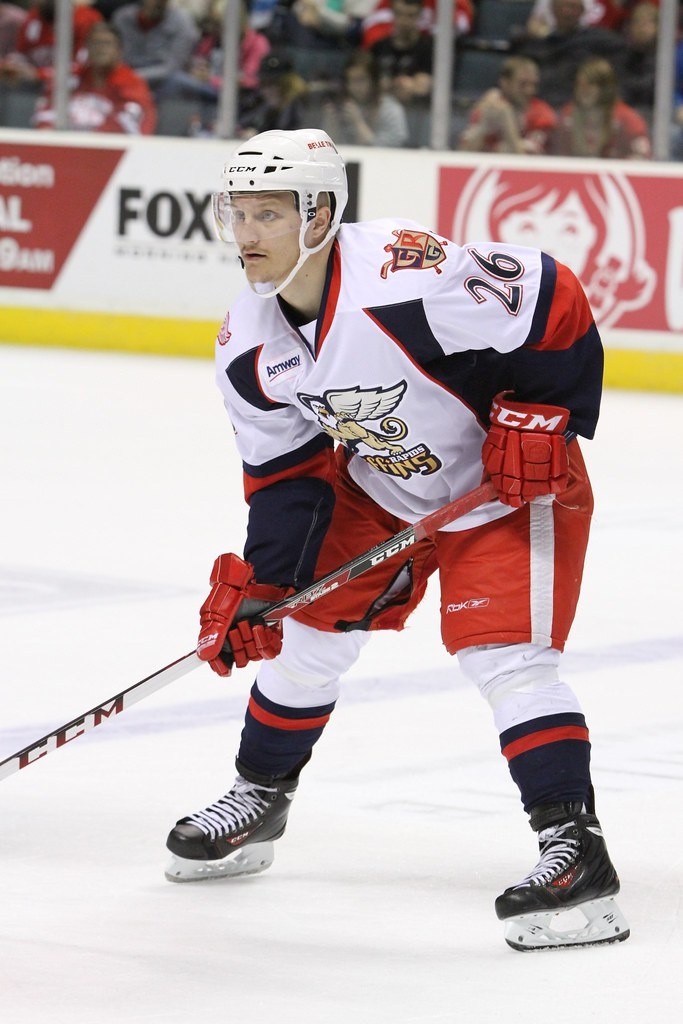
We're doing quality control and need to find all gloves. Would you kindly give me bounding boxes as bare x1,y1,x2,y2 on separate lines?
196,551,287,678
479,390,569,507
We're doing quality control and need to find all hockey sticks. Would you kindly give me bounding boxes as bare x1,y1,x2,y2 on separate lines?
0,479,503,770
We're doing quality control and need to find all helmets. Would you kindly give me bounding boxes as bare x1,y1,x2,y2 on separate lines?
211,129,348,299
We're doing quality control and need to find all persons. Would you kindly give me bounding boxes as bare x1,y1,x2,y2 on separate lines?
164,129,631,954
0,0,683,162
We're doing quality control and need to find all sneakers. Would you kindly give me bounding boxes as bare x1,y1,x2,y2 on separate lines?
494,800,636,953
163,758,300,882
